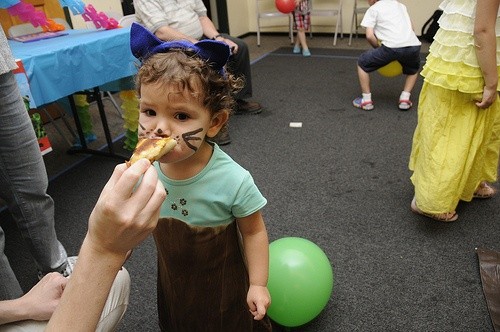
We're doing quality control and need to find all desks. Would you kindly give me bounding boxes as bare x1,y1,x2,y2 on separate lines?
0,27,142,211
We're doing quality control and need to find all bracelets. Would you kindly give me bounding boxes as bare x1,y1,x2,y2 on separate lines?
212,35,223,40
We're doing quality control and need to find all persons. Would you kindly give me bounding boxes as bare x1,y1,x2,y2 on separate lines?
0,159,167,332
132,0,262,115
408,0,500,222
352,0,418,111
0,24,79,300
292,0,312,56
130,49,271,332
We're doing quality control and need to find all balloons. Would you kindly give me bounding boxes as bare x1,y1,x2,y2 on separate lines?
275,0,297,14
263,237,333,327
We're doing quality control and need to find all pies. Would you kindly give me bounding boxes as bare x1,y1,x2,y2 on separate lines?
128,138,178,164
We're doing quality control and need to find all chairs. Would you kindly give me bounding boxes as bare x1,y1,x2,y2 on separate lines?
256,0,370,48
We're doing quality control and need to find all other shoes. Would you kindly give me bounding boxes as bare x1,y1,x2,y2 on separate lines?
232,99,262,114
410,195,459,222
293,44,302,53
63,257,78,276
353,98,374,110
399,100,413,109
213,132,231,145
303,48,311,56
473,183,496,198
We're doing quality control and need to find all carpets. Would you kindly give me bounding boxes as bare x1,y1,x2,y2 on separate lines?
3,44,500,332
